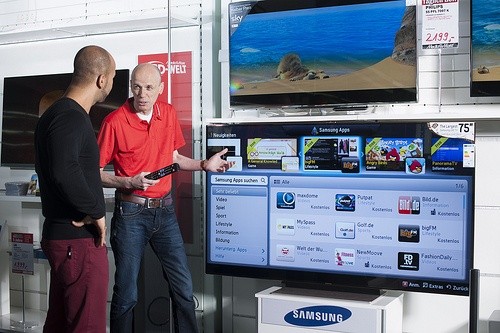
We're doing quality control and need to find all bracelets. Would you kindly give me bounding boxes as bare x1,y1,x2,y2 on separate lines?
200,160,206,171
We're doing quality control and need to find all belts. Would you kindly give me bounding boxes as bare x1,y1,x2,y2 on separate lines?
115,192,173,209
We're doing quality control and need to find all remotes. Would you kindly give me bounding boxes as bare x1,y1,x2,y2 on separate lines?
144,163,181,180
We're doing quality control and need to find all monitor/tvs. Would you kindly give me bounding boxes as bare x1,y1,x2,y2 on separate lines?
0,69,130,171
228,0,419,111
470,0,500,103
206,120,477,302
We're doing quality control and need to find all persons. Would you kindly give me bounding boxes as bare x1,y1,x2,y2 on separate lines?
33,46,115,333
97,64,236,333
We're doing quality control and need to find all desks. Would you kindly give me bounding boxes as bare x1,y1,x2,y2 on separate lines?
255,286,404,333
